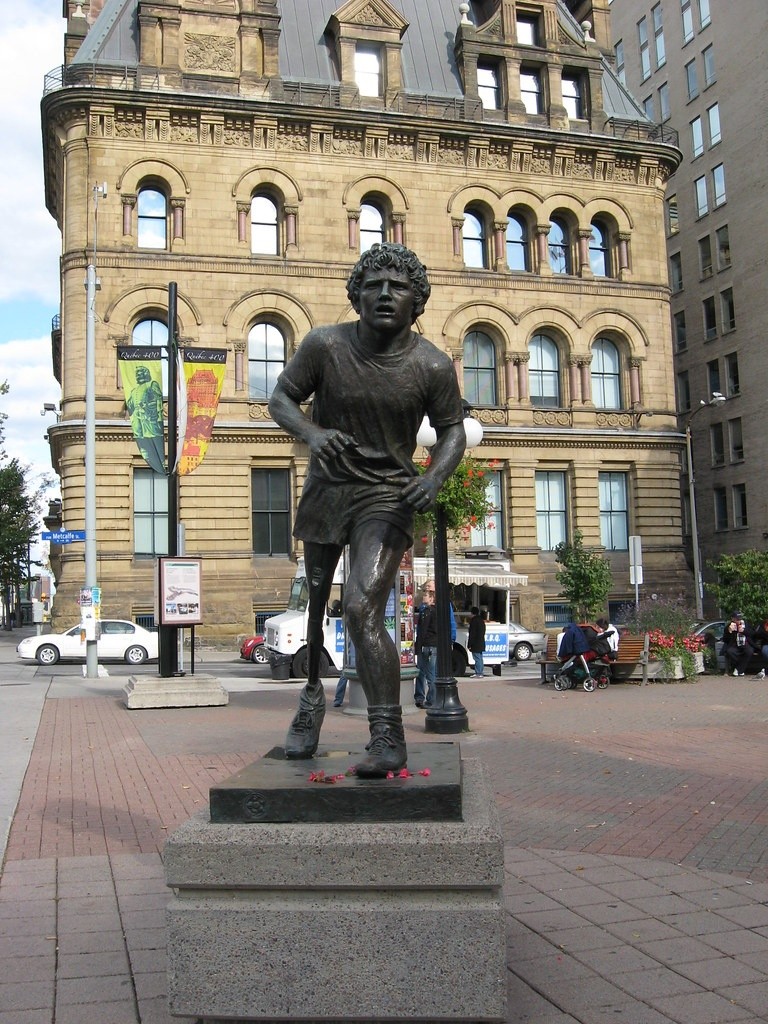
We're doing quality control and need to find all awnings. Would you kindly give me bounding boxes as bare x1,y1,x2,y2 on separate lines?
411,564,529,587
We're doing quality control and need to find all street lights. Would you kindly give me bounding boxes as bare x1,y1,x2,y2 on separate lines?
685,390,727,621
80,180,108,681
416,394,484,734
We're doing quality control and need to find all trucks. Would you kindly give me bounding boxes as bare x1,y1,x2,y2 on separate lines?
264,552,529,679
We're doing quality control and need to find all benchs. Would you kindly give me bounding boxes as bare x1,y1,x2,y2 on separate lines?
724,640,762,676
535,631,649,686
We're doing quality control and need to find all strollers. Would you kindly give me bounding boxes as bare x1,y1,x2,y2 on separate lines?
553,624,615,692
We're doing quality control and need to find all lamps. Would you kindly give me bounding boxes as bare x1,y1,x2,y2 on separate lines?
54,498,62,513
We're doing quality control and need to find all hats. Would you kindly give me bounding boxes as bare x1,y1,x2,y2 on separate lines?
733,611,743,619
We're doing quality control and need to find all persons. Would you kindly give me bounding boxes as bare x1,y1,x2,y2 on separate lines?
557,619,620,675
466,606,485,678
750,612,768,677
414,591,438,708
416,578,457,654
719,611,764,676
267,244,468,778
333,662,349,707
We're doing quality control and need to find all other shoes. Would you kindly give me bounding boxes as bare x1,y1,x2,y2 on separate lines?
423,704,430,708
470,673,476,677
476,675,483,678
740,672,745,675
334,704,343,707
733,668,738,676
415,700,423,708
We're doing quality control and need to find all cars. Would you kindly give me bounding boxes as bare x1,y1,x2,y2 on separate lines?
686,618,768,676
17,618,158,666
239,635,269,664
509,619,550,660
